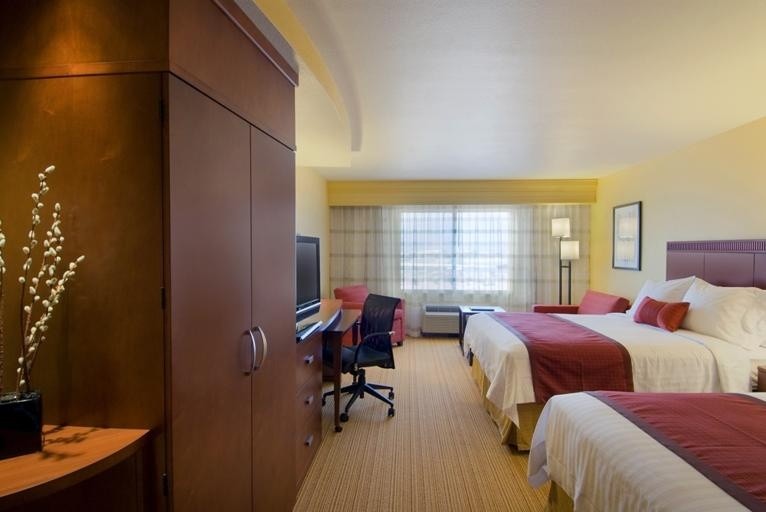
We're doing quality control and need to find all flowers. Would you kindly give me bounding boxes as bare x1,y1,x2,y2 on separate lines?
0,161,87,404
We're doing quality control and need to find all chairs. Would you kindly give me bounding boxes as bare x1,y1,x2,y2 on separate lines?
321,292,403,422
334,284,404,349
532,290,632,313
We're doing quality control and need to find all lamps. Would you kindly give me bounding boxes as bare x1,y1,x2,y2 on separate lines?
551,217,579,305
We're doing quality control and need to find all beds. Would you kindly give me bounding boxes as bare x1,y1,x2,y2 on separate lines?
523,386,765,511
461,238,766,453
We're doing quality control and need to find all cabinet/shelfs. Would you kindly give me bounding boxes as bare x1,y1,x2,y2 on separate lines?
290,325,328,499
0,1,300,509
1,425,157,511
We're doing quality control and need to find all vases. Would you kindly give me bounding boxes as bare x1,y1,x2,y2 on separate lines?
0,385,44,463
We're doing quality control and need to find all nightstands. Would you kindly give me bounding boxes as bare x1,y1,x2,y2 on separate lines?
756,362,766,390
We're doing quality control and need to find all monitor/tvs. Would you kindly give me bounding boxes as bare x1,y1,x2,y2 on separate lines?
297,236,321,335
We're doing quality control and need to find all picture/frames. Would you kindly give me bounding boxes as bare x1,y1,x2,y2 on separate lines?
611,199,643,271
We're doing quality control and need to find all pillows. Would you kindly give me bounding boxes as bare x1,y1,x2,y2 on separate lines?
679,274,766,350
624,273,696,319
633,294,693,332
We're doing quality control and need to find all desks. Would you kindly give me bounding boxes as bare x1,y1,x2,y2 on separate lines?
316,297,363,436
458,304,508,366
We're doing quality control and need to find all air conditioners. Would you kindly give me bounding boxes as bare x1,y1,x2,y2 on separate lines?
418,304,462,335
